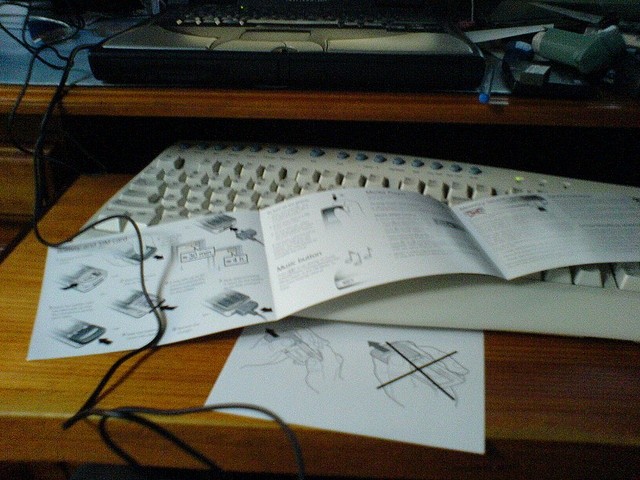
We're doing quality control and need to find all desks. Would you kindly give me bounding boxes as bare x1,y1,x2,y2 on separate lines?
0,83,638,478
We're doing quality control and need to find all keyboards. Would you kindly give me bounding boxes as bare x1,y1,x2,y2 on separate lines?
61,139,639,342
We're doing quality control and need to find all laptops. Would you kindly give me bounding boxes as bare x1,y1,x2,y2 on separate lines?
87,0,485,89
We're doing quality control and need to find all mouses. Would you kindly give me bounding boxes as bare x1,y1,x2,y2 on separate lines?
504,40,534,59
27,12,69,41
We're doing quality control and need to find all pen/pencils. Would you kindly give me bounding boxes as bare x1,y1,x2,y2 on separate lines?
479,63,495,104
